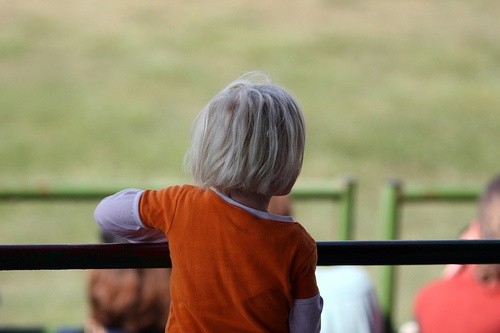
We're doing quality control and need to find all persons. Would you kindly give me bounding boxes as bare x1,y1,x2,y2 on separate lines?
92,72,324,333
416,172,500,333
